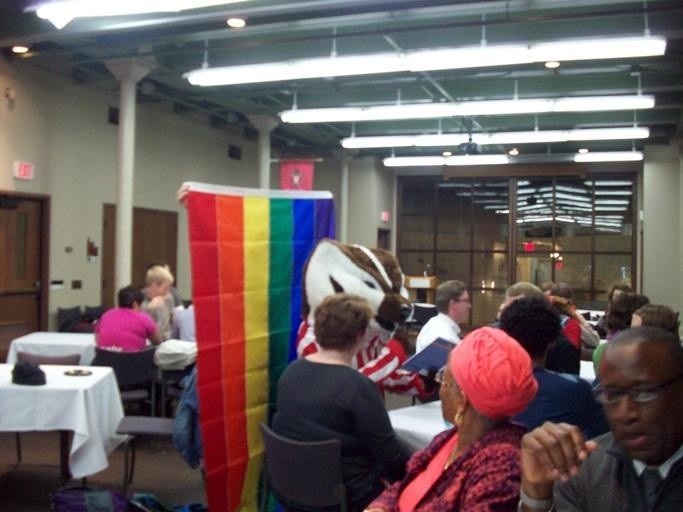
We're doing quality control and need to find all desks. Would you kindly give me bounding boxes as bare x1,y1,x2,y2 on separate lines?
576,308,605,326
1,362,134,492
6,330,197,372
579,357,597,384
380,399,455,458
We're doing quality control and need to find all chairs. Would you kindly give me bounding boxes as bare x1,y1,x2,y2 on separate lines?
84,305,107,313
256,422,347,512
14,348,208,495
57,306,80,328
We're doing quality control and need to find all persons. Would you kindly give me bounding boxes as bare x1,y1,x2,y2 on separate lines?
364,276,682,512
95,264,195,391
267,295,402,512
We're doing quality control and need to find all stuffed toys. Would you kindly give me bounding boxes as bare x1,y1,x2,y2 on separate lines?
294,238,440,402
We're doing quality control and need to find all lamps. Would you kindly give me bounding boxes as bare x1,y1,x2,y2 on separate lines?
432,180,634,236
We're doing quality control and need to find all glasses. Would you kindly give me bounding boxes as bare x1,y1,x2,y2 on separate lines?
434,366,459,390
591,378,675,405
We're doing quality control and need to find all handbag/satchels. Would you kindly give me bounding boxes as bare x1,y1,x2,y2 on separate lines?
52,484,207,512
